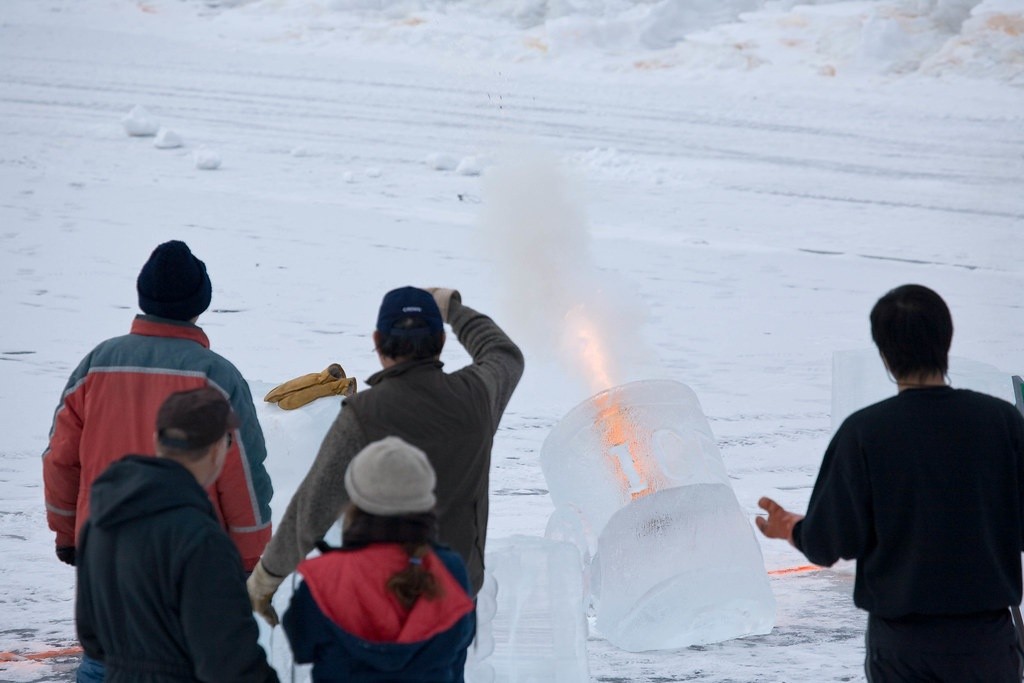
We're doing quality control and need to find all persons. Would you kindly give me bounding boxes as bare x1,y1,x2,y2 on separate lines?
41,240,274,577
75,388,282,683
282,435,474,683
247,286,524,628
756,282,1024,683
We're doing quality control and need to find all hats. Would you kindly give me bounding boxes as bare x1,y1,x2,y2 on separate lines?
343,436,437,516
136,240,212,318
377,287,443,336
156,387,241,448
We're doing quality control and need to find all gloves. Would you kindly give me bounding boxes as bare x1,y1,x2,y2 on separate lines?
244,560,286,625
423,288,461,324
754,497,807,552
264,363,356,410
